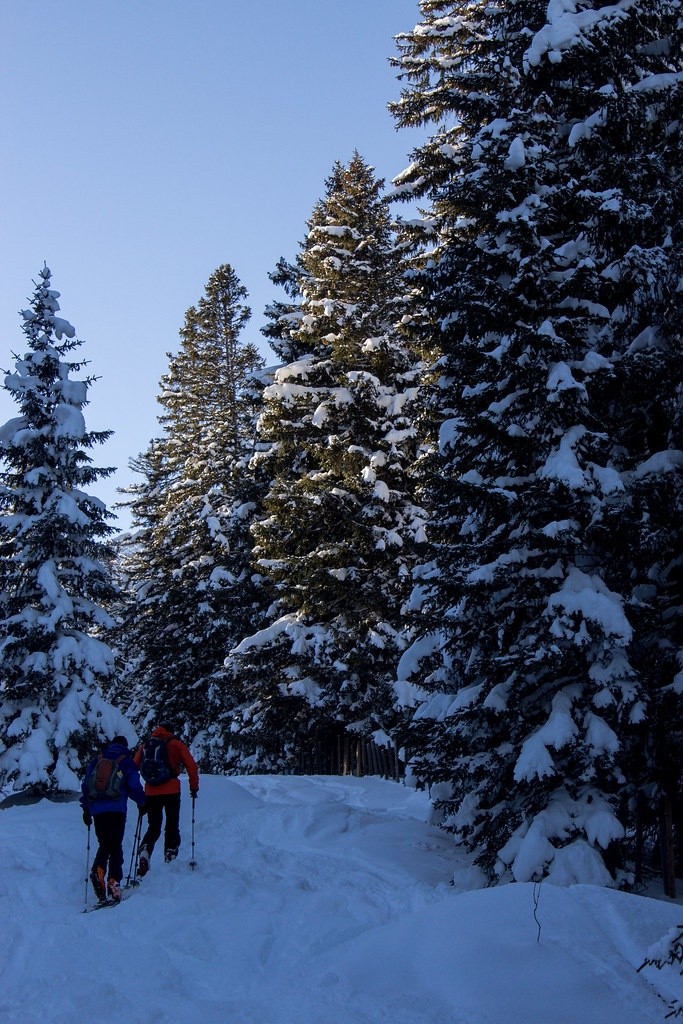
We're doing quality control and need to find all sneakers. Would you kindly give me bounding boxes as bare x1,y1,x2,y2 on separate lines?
107,878,121,902
90,867,107,901
139,844,150,876
165,848,179,862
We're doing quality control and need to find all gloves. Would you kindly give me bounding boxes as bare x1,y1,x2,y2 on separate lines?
190,790,199,799
83,811,93,826
137,803,147,816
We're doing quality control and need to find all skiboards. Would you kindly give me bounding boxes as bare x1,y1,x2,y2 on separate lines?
80,885,139,914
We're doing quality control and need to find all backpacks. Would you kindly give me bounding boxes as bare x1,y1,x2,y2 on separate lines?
87,753,127,800
140,736,179,786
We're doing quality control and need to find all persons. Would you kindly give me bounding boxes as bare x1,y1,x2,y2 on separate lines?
129,719,200,878
79,735,147,903
